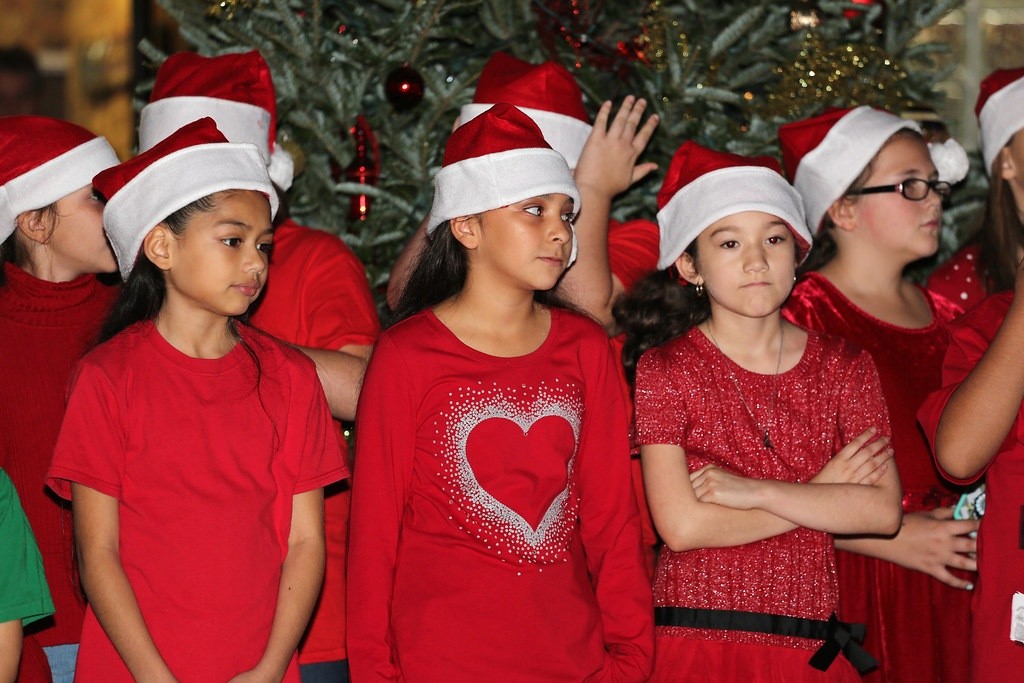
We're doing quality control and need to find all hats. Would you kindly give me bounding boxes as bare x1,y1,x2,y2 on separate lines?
654,139,813,271
138,47,294,192
0,114,121,246
452,50,596,170
974,67,1024,177
776,104,928,237
91,116,281,279
425,100,582,267
914,120,970,184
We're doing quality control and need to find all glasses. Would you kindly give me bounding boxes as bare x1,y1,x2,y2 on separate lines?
844,178,954,201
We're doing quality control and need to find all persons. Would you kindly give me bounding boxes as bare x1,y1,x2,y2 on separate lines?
773,104,983,683
0,43,384,683
386,51,660,339
914,67,1024,683
36,117,351,683
346,102,656,683
610,140,904,683
